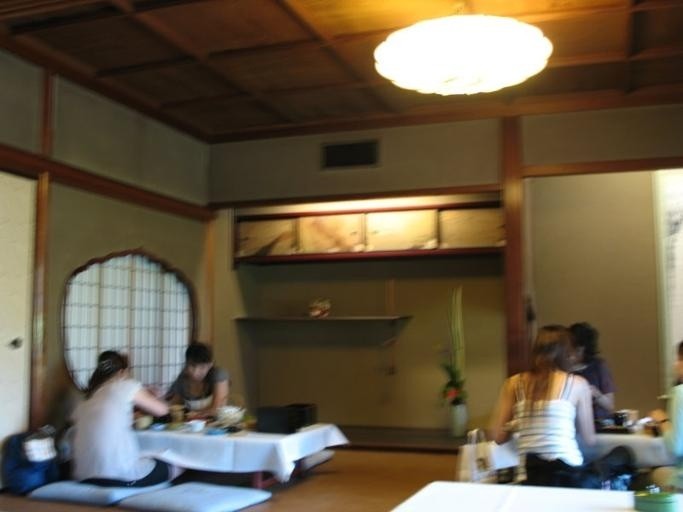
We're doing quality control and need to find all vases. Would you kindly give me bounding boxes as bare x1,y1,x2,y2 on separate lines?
450,404,467,437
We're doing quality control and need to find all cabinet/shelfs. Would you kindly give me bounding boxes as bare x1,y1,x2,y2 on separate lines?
233,200,505,270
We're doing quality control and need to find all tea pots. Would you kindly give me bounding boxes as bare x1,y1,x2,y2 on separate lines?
216,393,247,424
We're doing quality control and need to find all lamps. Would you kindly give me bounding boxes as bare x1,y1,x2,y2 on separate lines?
374,0,554,97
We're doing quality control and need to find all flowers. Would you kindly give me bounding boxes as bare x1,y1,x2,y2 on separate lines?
438,360,470,407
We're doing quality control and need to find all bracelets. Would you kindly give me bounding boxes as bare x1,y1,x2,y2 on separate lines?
657,417,671,424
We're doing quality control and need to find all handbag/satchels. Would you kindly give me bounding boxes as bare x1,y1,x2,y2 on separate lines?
457,428,497,483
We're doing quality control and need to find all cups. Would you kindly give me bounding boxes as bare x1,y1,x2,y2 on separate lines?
190,419,206,433
614,409,634,427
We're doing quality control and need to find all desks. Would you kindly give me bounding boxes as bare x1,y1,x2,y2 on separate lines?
132,419,349,490
458,434,669,483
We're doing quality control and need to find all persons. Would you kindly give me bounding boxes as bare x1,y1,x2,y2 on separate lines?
66,348,185,488
481,322,633,488
161,341,230,420
647,340,682,465
560,321,617,422
